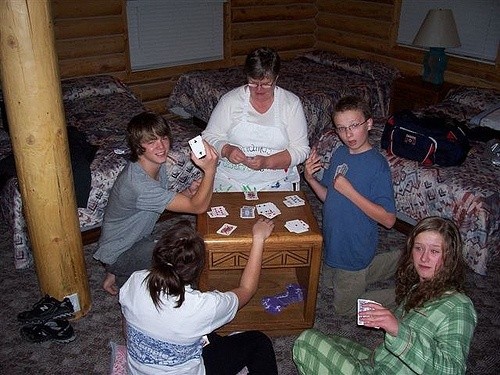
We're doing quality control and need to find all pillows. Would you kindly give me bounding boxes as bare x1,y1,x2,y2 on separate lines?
302,50,397,79
59,75,128,102
442,88,500,110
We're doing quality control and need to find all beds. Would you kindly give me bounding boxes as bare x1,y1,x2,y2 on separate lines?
166,47,404,175
310,85,500,277
0,74,205,271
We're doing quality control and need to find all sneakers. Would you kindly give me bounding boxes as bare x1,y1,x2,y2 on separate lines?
19,320,76,344
17,294,75,323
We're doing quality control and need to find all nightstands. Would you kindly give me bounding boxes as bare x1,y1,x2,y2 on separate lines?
387,75,458,118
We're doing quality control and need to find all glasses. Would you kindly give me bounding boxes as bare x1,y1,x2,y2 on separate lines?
246,76,277,89
336,118,369,134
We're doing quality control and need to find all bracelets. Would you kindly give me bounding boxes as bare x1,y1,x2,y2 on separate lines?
187,187,192,195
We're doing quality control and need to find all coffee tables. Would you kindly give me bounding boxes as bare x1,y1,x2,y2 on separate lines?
196,191,322,338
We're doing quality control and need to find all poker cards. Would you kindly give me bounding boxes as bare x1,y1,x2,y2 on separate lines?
283,194,306,208
239,206,256,219
188,135,207,159
284,219,309,233
244,190,259,201
216,223,237,236
357,298,383,329
255,201,282,220
206,205,230,219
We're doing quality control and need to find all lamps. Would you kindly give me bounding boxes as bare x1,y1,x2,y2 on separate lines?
411,8,462,85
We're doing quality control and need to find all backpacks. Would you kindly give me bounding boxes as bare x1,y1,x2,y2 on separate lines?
381,108,474,168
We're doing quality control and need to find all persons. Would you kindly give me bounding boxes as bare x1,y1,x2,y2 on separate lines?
199,46,311,192
292,215,478,375
304,95,405,319
93,112,220,294
118,220,279,375
